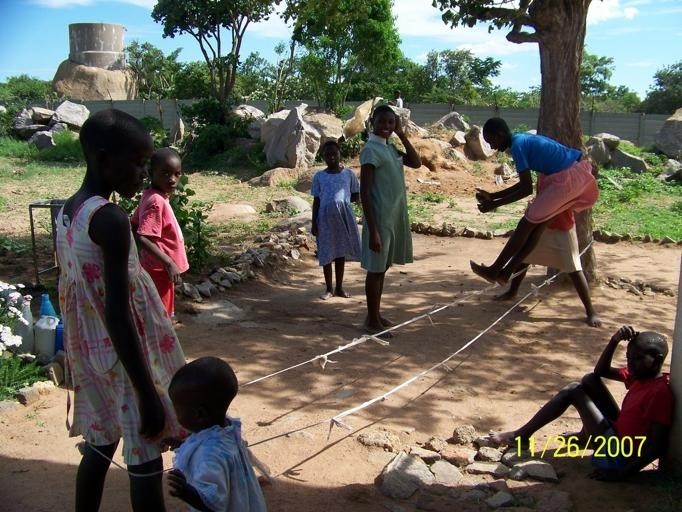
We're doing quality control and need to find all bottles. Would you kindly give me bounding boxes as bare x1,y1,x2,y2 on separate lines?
14,294,60,359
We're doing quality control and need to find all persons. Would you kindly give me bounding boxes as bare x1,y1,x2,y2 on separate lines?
310,140,360,300
490,211,601,328
359,104,421,338
469,118,600,286
131,147,189,317
56,108,189,511
388,90,404,108
488,324,671,483
161,355,267,511
